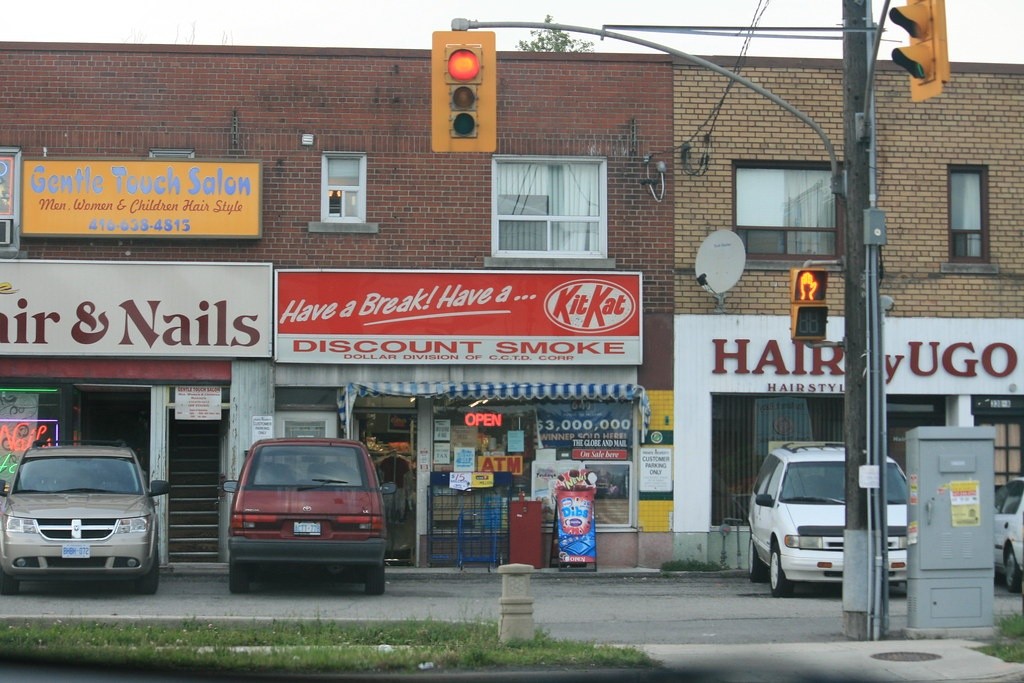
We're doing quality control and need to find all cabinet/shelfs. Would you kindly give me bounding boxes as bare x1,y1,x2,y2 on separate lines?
509,501,546,568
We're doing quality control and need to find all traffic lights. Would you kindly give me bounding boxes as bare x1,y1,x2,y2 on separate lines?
888,0,935,86
444,44,484,138
789,267,830,342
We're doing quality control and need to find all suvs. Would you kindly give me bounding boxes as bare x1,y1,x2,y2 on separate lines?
0,440,170,595
747,440,907,598
221,437,398,596
995,477,1024,592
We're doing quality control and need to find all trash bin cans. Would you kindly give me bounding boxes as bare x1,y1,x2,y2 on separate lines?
508,501,542,570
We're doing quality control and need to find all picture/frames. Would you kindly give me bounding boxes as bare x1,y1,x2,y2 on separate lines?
387,414,417,434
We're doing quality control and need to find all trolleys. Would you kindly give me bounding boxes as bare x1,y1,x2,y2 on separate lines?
459,487,499,572
